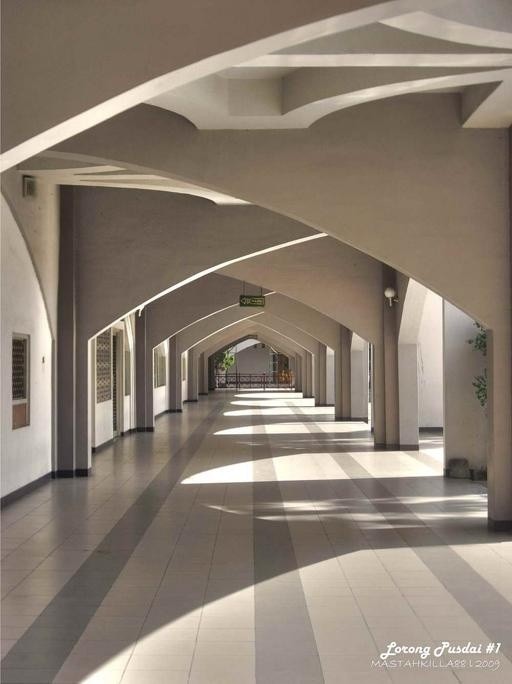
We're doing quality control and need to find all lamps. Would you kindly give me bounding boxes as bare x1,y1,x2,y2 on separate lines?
384,286,400,308
